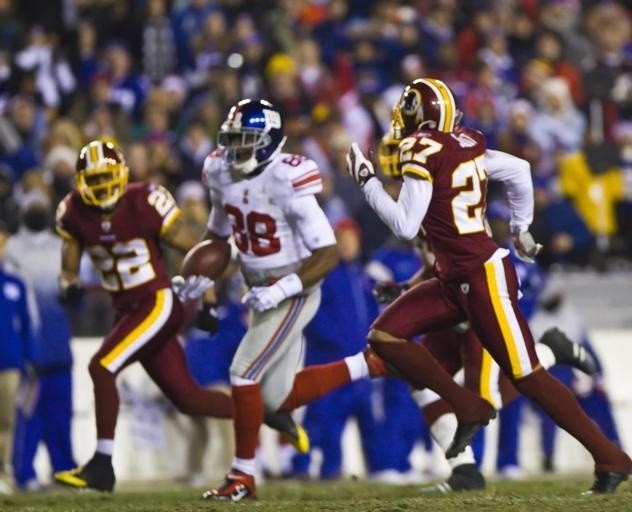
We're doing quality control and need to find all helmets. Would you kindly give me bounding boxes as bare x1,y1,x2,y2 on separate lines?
215,97,287,180
391,76,465,142
377,130,407,181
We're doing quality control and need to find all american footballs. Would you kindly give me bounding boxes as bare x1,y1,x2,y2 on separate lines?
181,237,232,279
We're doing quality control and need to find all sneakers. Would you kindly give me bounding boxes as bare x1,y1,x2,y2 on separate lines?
538,326,598,377
363,342,426,393
200,468,259,505
418,467,487,495
262,409,310,456
444,400,497,462
50,451,116,496
580,450,631,501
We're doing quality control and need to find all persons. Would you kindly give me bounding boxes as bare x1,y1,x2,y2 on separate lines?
288,0,392,259
393,1,463,102
0,189,42,497
209,0,289,113
7,192,79,494
127,0,208,219
54,139,309,492
345,77,632,500
0,0,128,199
172,98,401,501
374,130,597,495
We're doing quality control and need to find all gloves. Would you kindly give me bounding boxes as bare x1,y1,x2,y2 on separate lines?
240,273,306,314
344,141,383,195
196,300,220,339
168,273,217,306
64,285,90,304
508,223,544,265
74,139,129,213
